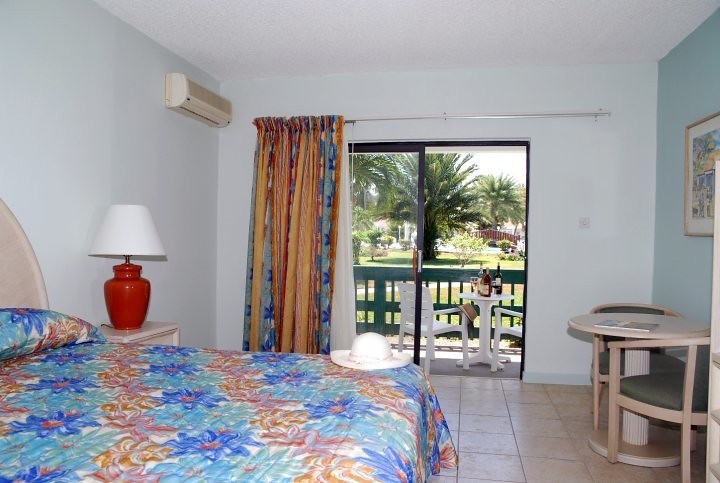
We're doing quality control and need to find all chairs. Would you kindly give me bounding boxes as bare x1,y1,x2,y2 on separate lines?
396,283,469,376
590,303,683,432
491,306,523,372
608,336,712,483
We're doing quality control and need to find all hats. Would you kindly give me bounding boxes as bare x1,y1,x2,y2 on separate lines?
454,300,477,322
329,332,412,371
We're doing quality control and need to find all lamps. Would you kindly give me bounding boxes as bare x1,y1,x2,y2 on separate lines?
88,204,168,331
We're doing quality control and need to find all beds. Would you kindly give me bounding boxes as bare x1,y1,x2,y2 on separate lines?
0,198,460,483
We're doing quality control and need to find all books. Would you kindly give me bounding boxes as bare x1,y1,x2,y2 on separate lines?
593,320,659,333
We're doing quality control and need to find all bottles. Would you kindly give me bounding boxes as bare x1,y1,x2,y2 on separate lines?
483,268,491,297
495,262,503,294
480,269,486,296
478,262,484,295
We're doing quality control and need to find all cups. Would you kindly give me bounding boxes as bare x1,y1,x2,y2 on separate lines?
471,277,478,295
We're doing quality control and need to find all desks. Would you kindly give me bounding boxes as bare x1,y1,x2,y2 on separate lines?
568,313,710,468
457,293,514,370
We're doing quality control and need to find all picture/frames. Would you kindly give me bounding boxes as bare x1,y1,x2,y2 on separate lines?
684,111,720,237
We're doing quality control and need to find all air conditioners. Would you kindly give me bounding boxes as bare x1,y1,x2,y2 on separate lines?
165,72,232,128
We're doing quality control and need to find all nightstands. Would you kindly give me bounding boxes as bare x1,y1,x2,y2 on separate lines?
97,320,180,347
